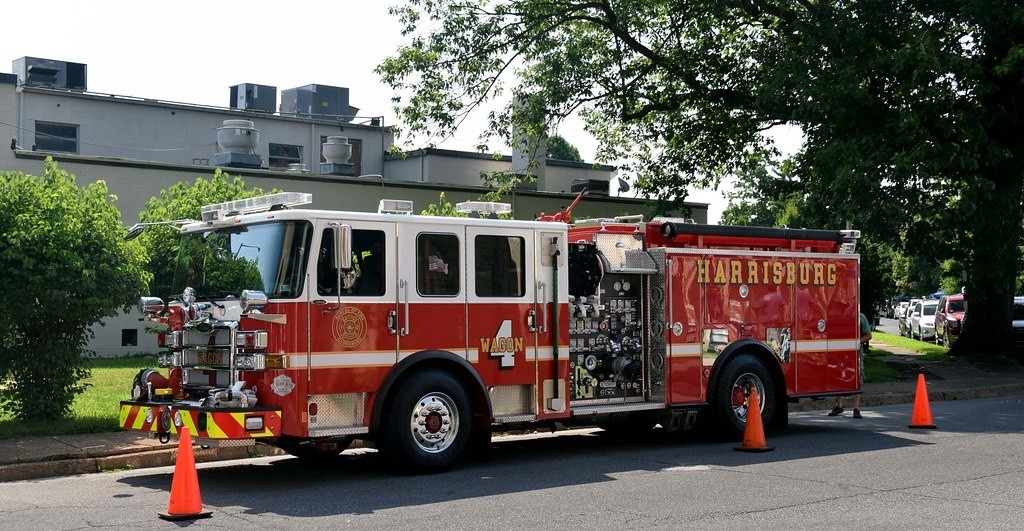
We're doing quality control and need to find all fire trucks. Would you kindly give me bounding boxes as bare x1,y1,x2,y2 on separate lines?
118,185,862,478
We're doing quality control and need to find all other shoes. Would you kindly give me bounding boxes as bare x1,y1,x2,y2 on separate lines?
853,409,862,419
829,405,844,416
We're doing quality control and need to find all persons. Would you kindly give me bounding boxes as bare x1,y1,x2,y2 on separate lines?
829,313,872,419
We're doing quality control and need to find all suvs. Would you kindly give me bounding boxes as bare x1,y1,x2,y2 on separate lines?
934,294,967,347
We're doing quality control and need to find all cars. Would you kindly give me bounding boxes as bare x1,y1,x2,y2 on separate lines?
1011,297,1024,333
894,301,909,319
898,299,925,338
909,300,939,342
876,299,899,319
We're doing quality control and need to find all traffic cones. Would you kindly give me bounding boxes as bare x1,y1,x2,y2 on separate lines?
157,427,214,521
907,373,937,429
733,388,777,452
